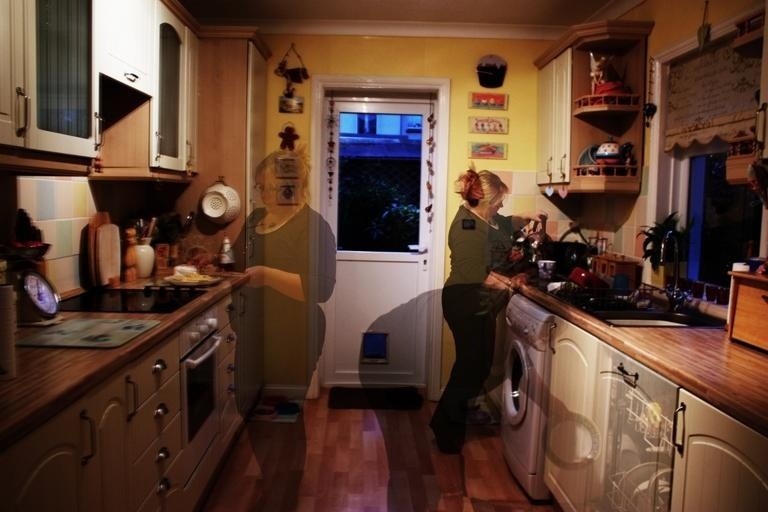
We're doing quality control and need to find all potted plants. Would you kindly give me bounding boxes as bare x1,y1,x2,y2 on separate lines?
635,209,697,289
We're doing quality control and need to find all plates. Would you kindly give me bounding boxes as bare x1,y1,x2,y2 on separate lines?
162,275,222,287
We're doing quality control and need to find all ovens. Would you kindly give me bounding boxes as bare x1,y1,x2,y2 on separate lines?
175,306,225,509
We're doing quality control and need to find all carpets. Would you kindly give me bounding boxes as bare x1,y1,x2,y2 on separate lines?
329,385,424,412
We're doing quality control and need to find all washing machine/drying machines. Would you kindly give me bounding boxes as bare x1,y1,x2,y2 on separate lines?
501,294,557,502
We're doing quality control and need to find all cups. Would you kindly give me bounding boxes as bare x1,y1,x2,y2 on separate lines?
537,259,556,279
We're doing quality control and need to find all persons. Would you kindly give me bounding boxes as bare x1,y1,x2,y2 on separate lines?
359,270,574,511
190,146,338,510
430,168,549,453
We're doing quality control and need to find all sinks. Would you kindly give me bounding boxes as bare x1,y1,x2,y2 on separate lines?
592,308,722,327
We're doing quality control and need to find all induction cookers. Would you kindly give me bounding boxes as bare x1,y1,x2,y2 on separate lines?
55,287,206,315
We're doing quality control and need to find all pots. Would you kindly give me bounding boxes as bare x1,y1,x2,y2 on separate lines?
539,241,587,271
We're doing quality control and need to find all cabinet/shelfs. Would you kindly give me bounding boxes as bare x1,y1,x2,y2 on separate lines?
149,1,202,176
0,0,104,164
529,20,655,201
96,2,150,177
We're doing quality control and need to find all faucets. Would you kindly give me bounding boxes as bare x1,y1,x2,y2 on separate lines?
659,231,693,313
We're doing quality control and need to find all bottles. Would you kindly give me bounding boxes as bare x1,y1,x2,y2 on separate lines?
219,238,235,265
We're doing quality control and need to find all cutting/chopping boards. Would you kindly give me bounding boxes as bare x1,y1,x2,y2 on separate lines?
87,211,122,287
17,315,159,351
